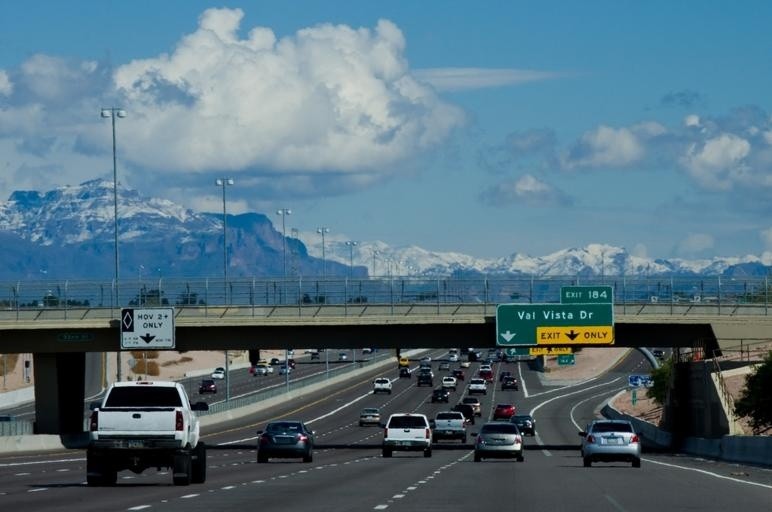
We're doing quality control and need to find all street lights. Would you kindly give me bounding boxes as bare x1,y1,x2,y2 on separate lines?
344,240,357,303
371,249,448,303
316,225,329,303
215,177,235,302
599,248,606,285
99,103,128,307
276,206,291,304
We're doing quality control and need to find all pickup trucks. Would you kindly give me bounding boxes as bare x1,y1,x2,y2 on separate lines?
85,379,207,485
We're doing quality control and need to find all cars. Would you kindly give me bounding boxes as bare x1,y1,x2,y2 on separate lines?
304,350,535,462
577,416,642,468
253,351,296,377
199,378,216,393
257,417,315,463
212,367,225,380
654,350,665,359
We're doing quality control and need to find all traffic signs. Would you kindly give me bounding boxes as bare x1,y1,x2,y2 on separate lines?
560,286,612,303
558,354,576,365
495,303,615,346
630,376,652,387
120,307,175,351
506,347,571,354
